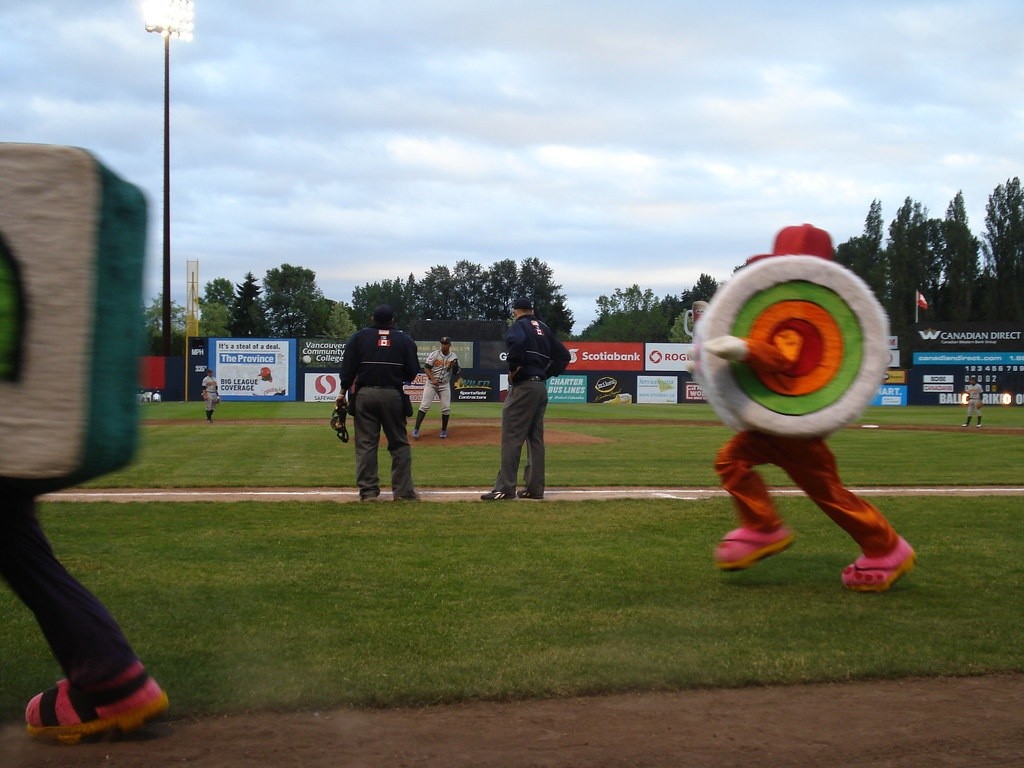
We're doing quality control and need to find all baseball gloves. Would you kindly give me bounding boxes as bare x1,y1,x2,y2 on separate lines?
329,394,349,444
450,358,460,375
212,396,220,404
976,400,983,409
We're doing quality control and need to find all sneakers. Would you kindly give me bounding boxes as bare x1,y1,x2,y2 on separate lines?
517,488,543,501
480,489,518,501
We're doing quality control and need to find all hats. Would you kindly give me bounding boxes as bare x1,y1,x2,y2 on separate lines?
439,337,450,343
372,303,395,326
207,369,212,372
511,296,533,309
258,367,269,378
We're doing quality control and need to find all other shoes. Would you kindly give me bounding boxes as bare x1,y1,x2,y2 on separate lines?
412,428,419,439
961,424,969,427
394,488,420,501
360,493,378,503
975,424,981,428
440,429,447,438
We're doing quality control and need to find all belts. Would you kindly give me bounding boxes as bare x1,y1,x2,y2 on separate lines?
363,385,397,389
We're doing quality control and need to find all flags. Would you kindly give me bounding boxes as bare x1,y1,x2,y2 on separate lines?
917,291,928,309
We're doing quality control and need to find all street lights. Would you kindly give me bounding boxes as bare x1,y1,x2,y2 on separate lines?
144,0,195,357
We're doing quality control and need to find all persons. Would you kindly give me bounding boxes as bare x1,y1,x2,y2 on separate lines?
480,296,572,500
691,224,917,593
961,377,984,428
138,385,145,394
0,144,167,744
154,386,160,394
335,304,420,502
411,336,459,439
201,369,219,423
